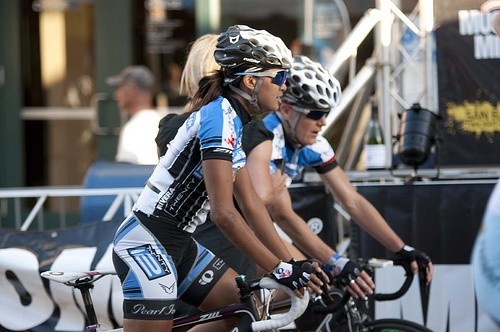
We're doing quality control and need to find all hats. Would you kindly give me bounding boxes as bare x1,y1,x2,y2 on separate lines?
104,64,157,91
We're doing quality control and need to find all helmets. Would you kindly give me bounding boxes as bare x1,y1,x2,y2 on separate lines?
280,54,343,111
213,23,293,76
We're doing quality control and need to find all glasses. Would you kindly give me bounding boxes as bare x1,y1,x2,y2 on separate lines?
288,105,330,122
231,68,290,87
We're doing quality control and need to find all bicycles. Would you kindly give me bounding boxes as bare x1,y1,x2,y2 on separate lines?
258,249,437,332
34,261,328,332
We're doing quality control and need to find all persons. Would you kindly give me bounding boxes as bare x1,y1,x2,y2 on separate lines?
154,34,221,161
193,55,434,314
112,24,331,332
106,65,166,166
